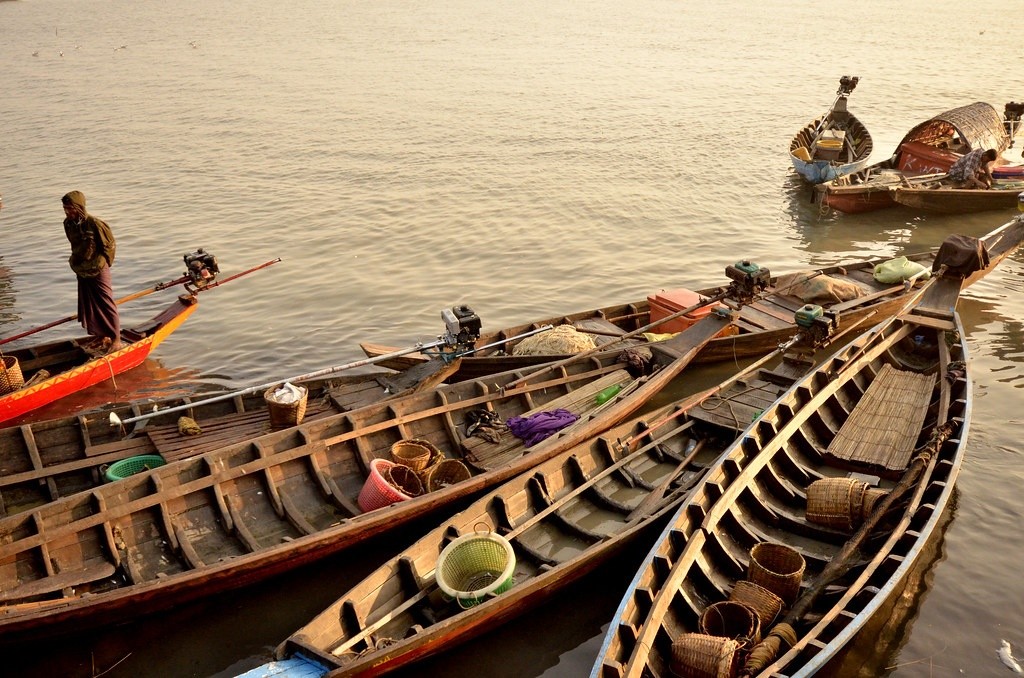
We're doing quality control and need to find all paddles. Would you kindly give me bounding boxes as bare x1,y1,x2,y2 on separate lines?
623,436,711,523
0,558,116,607
828,275,928,313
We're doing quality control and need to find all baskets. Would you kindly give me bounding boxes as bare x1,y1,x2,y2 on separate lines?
107,455,166,482
423,458,472,493
0,356,24,395
358,458,414,513
702,601,754,640
391,443,431,471
699,600,762,650
805,476,890,532
435,522,516,612
262,382,309,428
383,463,423,497
748,541,806,606
670,633,730,678
728,580,786,629
388,438,445,475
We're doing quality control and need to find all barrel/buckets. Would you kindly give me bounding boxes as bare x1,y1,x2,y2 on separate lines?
816,140,842,161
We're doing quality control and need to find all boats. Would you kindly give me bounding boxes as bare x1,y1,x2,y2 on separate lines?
585,234,989,678
0,246,219,426
0,244,945,678
812,101,1024,215
891,163,1024,215
786,73,875,187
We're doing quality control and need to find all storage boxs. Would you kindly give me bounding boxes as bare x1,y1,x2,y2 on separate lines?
646,288,733,337
823,130,845,143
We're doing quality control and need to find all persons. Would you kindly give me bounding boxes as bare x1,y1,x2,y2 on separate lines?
60,190,123,354
950,148,998,189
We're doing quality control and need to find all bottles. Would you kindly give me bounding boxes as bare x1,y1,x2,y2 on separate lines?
596,383,623,405
